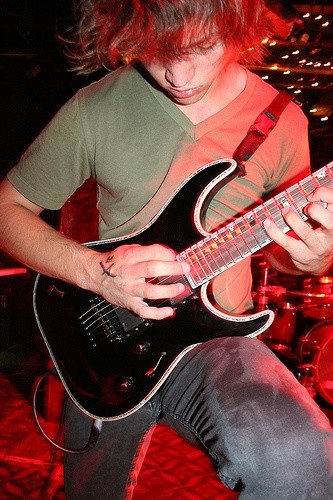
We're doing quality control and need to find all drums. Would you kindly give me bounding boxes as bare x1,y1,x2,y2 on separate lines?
294,322,333,405
301,274,333,322
249,282,296,353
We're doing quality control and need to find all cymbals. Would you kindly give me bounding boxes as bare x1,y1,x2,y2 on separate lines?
287,286,333,308
251,249,269,262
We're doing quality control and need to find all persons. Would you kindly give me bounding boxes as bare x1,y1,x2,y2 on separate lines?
0,0,333,499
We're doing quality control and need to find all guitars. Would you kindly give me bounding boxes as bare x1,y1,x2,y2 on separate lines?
21,158,333,422
